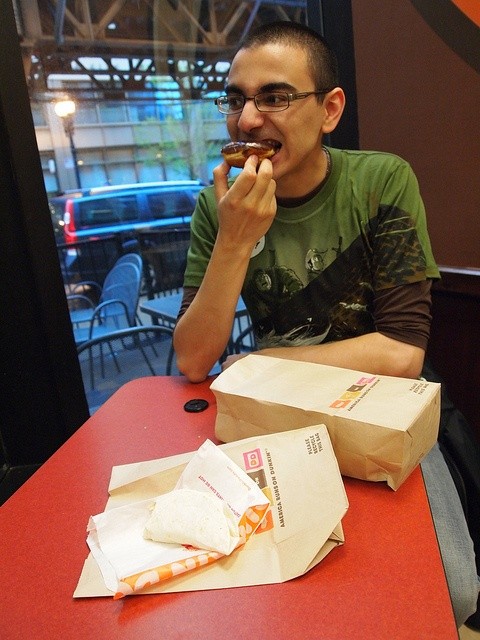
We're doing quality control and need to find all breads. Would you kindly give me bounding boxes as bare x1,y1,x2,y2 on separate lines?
221,142,275,167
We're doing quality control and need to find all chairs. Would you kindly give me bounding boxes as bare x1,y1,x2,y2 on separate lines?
72,262,158,390
67,254,157,375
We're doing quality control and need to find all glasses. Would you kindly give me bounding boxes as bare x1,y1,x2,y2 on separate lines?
215,88,332,115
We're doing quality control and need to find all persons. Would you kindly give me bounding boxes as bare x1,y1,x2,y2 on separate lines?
172,21,442,384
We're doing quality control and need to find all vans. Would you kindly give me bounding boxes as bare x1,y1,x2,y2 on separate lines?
51,179,219,298
